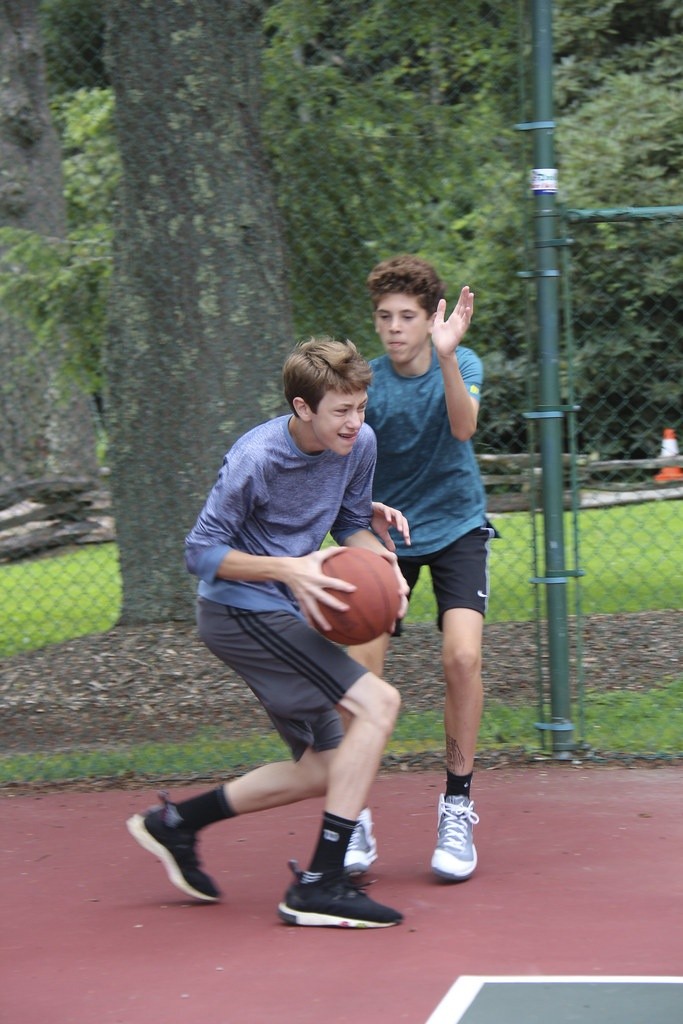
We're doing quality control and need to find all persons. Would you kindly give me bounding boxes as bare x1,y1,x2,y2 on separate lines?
125,335,412,931
331,257,501,881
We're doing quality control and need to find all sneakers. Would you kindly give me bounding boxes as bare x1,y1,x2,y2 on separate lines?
342,806,378,876
125,791,221,902
430,793,479,882
277,860,404,928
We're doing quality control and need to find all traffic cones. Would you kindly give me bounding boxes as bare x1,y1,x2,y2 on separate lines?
653,428,683,481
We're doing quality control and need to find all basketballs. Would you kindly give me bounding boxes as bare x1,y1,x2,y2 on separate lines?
306,547,401,645
